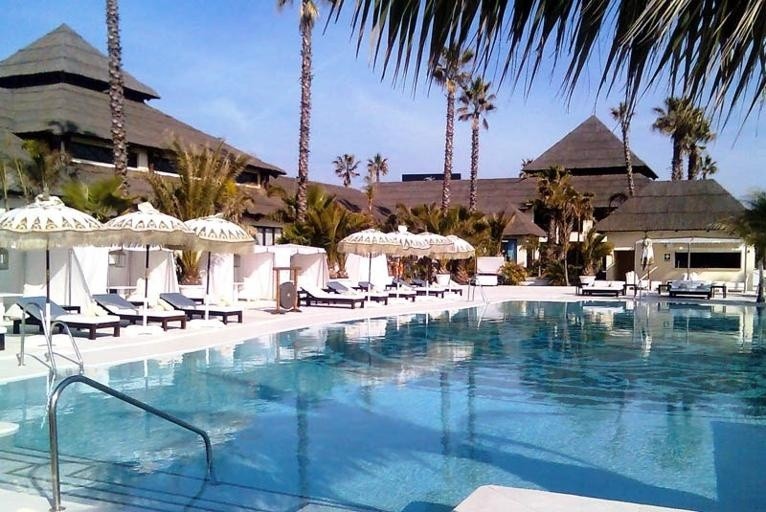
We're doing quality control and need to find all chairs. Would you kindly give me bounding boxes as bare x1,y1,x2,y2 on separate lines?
0,290,243,347
299,278,463,309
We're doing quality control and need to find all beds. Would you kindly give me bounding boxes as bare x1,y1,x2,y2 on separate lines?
576,277,726,298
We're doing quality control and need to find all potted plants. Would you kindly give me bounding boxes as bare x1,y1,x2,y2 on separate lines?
129,134,257,299
575,228,615,287
426,202,469,285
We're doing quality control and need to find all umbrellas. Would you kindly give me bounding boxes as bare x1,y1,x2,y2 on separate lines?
335,310,474,390
387,223,431,299
416,227,455,295
639,236,655,290
16,350,253,475
93,200,195,327
337,227,403,303
165,212,256,321
1,183,105,337
435,234,476,292
640,305,653,364
